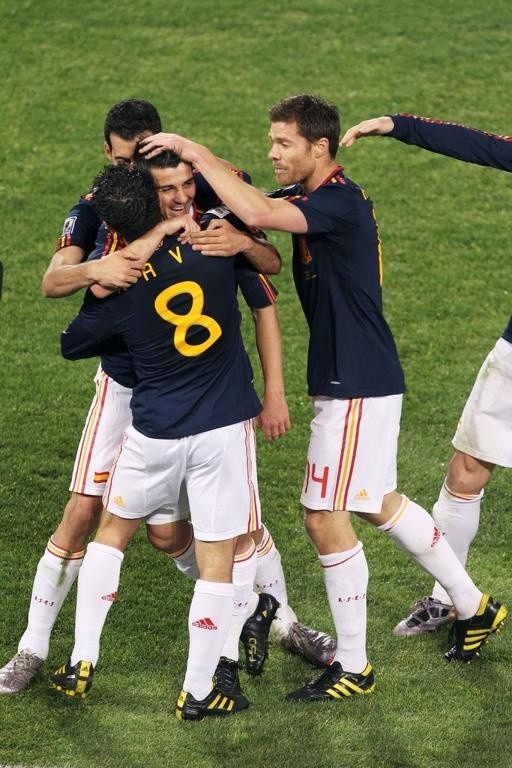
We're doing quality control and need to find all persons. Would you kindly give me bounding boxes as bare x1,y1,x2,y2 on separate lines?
139,96,511,703
1,99,337,726
341,107,511,637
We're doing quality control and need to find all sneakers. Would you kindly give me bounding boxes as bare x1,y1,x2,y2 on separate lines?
280,622,337,666
0,647,45,695
213,656,240,696
444,592,509,663
285,660,375,701
393,596,456,636
175,686,249,721
240,593,279,675
48,657,94,698
